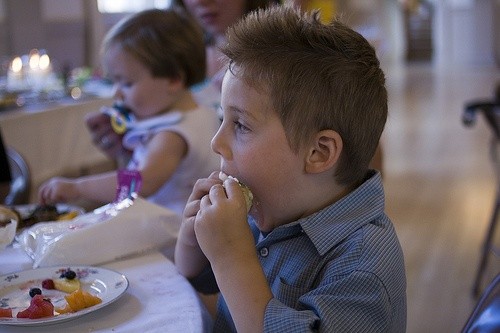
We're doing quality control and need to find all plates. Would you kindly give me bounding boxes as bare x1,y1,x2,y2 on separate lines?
0,265,130,325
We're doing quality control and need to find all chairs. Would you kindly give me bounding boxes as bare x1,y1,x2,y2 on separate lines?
461,273,500,333
0,145,30,205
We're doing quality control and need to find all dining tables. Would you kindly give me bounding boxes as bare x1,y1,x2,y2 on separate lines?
0,207,213,333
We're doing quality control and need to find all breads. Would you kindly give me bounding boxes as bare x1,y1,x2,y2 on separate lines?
218,170,254,217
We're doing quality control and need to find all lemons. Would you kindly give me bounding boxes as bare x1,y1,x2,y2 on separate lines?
52,277,80,293
